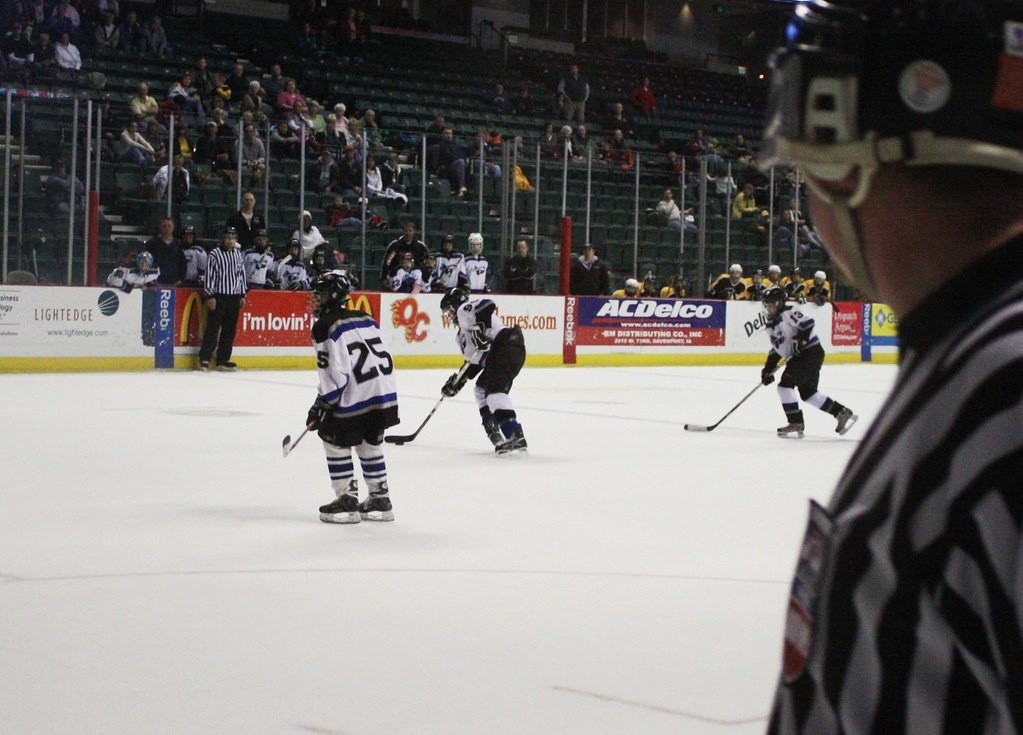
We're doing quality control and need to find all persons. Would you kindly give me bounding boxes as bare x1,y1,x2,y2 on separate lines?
761,287,858,439
0,0,493,295
569,242,608,296
199,225,247,372
613,264,831,306
440,289,529,454
762,0,1023,735
306,273,400,524
501,239,537,294
477,61,824,255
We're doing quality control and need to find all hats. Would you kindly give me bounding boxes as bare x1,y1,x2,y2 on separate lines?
581,243,594,252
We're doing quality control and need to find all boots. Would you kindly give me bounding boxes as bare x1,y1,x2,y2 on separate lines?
481,413,528,460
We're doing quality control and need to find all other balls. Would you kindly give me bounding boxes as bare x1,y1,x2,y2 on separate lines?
396,441,404,445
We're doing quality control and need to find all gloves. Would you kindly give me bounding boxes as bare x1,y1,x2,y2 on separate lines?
790,335,805,356
441,373,467,398
762,367,776,386
470,322,491,351
307,393,334,431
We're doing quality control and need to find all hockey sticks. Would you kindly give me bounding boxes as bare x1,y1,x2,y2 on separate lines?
684,352,794,432
384,347,480,443
281,419,315,458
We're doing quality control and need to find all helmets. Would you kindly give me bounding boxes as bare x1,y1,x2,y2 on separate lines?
222,227,238,238
181,226,195,236
814,270,826,280
439,289,471,323
625,279,639,295
468,233,484,254
769,264,780,273
441,234,457,253
289,239,299,256
313,249,328,268
760,287,786,315
313,270,351,318
729,264,742,281
757,0,1023,183
254,230,268,251
400,251,415,264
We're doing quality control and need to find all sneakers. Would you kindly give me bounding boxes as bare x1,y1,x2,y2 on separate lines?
776,421,805,439
834,406,858,435
317,491,361,523
356,495,394,521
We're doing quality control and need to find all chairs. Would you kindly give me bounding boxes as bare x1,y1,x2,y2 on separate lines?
0,8,857,302
